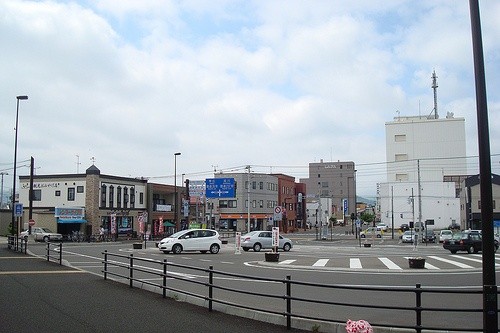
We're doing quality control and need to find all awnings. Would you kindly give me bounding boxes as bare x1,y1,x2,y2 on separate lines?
57,218,88,223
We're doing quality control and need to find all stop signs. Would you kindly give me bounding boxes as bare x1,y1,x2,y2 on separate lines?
29,219,35,226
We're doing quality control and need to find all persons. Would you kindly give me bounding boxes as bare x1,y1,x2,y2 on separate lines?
309,222,312,229
211,225,215,230
97,225,104,242
189,233,195,238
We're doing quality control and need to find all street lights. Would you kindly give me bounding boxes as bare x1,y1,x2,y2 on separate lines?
174,152,181,223
11,95,29,236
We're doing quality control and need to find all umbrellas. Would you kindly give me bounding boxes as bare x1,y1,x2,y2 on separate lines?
157,221,175,231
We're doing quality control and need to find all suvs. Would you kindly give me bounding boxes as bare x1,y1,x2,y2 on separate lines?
421,229,436,243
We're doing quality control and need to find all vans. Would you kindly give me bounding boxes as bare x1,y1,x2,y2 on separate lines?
414,222,424,232
157,228,222,254
461,230,482,240
438,230,453,243
359,227,383,238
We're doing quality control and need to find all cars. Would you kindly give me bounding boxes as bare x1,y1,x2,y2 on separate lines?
442,232,499,254
19,226,63,244
240,230,293,252
402,231,418,243
376,223,388,232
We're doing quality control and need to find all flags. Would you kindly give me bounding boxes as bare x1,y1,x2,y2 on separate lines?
138,219,145,238
